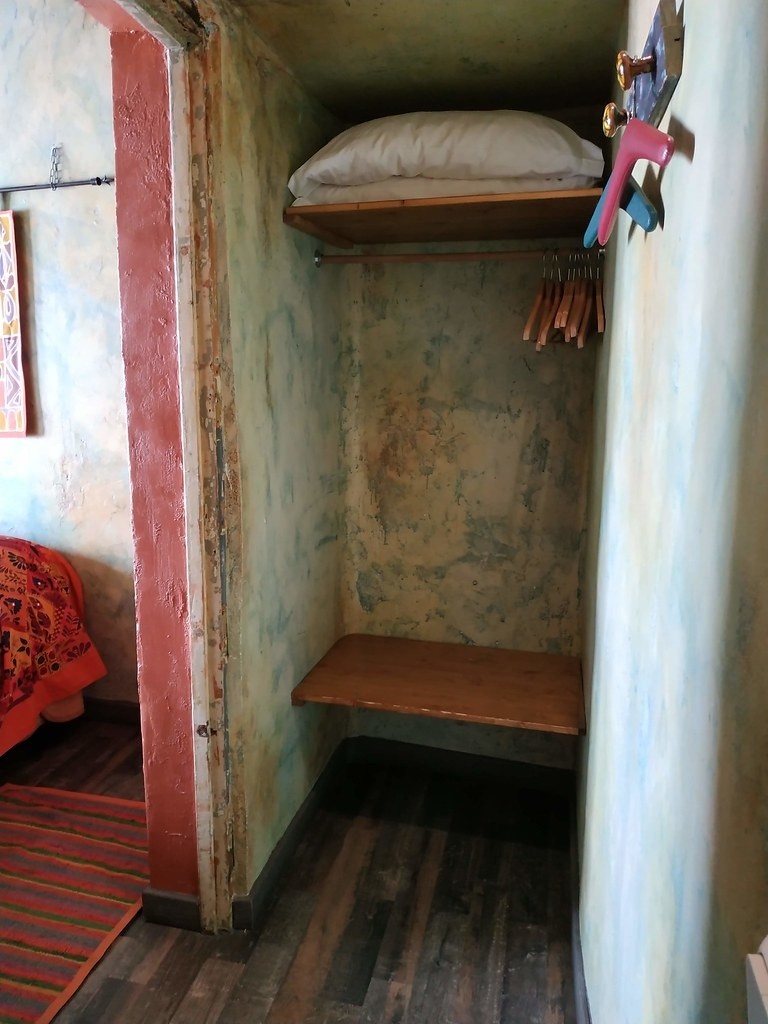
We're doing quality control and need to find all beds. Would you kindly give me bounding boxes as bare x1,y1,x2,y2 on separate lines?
0,534,107,768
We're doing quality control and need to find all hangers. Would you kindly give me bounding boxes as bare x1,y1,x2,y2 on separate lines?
521,246,606,353
596,55,676,247
582,106,660,248
